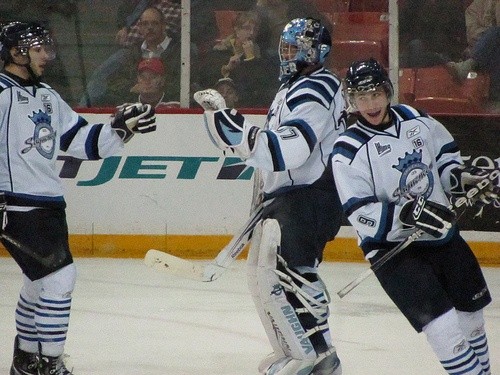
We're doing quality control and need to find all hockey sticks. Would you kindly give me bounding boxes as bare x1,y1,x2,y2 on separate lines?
337,168,500,300
143,105,354,283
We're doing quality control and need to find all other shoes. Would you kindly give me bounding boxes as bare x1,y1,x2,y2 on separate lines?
9,336,76,375
258,348,342,375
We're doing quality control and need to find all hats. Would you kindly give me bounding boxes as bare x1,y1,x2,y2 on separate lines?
138,58,165,75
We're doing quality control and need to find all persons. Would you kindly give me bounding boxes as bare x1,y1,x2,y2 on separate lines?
190,11,268,106
0,22,157,375
189,0,334,106
332,57,492,375
123,58,180,107
215,77,240,107
77,0,219,108
192,18,348,374
399,0,475,68
447,0,500,113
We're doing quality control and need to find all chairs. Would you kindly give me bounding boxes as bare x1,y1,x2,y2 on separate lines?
198,0,491,113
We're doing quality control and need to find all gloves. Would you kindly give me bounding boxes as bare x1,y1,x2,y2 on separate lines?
401,191,456,240
110,102,157,144
194,88,229,112
450,161,500,209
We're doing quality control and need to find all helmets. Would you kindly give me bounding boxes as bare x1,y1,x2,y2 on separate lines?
0,20,57,66
345,58,394,109
278,17,333,83
